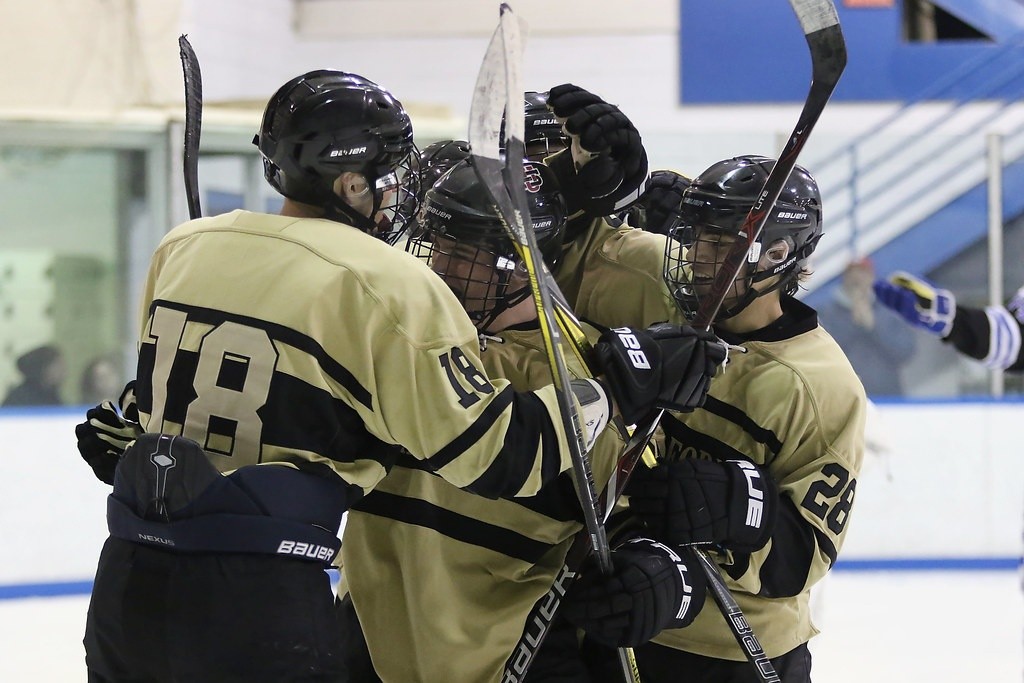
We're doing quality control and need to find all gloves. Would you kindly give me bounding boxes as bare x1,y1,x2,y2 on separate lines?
594,323,727,426
619,170,693,234
550,83,654,218
559,529,705,648
874,271,957,341
629,457,774,552
75,400,143,485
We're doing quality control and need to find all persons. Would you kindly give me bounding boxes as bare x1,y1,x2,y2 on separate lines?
73,69,867,683
816,258,1024,399
4,346,66,406
82,354,123,402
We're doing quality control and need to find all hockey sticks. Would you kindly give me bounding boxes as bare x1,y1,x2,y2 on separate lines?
179,35,204,227
469,1,849,682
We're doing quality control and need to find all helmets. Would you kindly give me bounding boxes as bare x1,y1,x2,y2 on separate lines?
405,155,567,334
662,154,824,326
400,140,473,240
499,91,596,241
258,68,424,247
